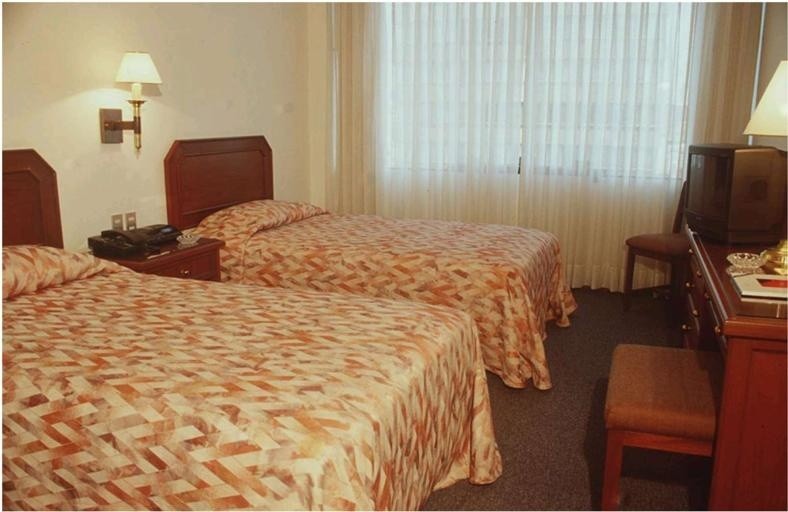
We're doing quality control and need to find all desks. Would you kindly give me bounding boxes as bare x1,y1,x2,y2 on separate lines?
682,220,787,511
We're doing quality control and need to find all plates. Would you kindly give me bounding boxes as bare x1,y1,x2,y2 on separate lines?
176,234,201,245
176,243,199,249
725,265,765,277
727,252,765,269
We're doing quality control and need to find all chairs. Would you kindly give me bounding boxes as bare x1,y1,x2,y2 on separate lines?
622,180,709,311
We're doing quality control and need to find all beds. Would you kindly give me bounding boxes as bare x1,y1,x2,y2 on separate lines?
165,136,579,390
2,148,505,512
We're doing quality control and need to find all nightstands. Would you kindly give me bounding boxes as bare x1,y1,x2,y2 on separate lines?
88,234,226,282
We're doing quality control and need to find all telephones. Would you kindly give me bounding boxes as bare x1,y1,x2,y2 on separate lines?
87,227,145,259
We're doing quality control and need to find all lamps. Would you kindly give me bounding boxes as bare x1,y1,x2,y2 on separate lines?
100,51,163,150
741,59,788,274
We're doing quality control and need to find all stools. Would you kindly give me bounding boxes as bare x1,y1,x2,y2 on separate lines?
601,341,724,511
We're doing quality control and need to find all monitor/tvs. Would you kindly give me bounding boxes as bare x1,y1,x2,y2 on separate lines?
683,143,788,244
